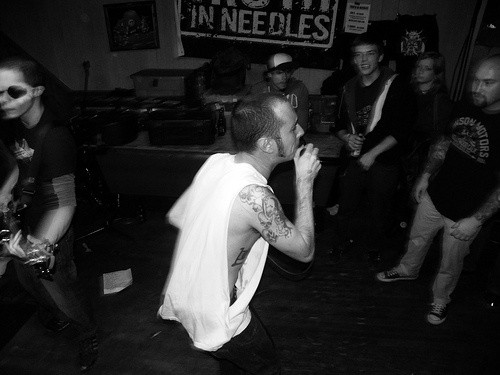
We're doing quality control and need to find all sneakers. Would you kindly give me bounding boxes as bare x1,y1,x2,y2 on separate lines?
427,304,450,325
377,268,419,282
47,316,69,332
78,334,97,372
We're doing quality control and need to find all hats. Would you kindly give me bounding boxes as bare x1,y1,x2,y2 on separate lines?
267,53,297,71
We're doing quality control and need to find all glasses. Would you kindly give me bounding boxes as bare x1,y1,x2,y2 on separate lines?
0,86,29,99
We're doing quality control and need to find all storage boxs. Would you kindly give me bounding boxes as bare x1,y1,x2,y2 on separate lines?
129,69,196,97
148,113,215,147
93,116,139,146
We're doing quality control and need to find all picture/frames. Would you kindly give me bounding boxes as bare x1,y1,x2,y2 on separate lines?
102,0,160,52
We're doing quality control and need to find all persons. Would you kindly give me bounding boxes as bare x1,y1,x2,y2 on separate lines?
160,91,322,373
0,60,115,375
240,32,464,264
375,58,496,324
